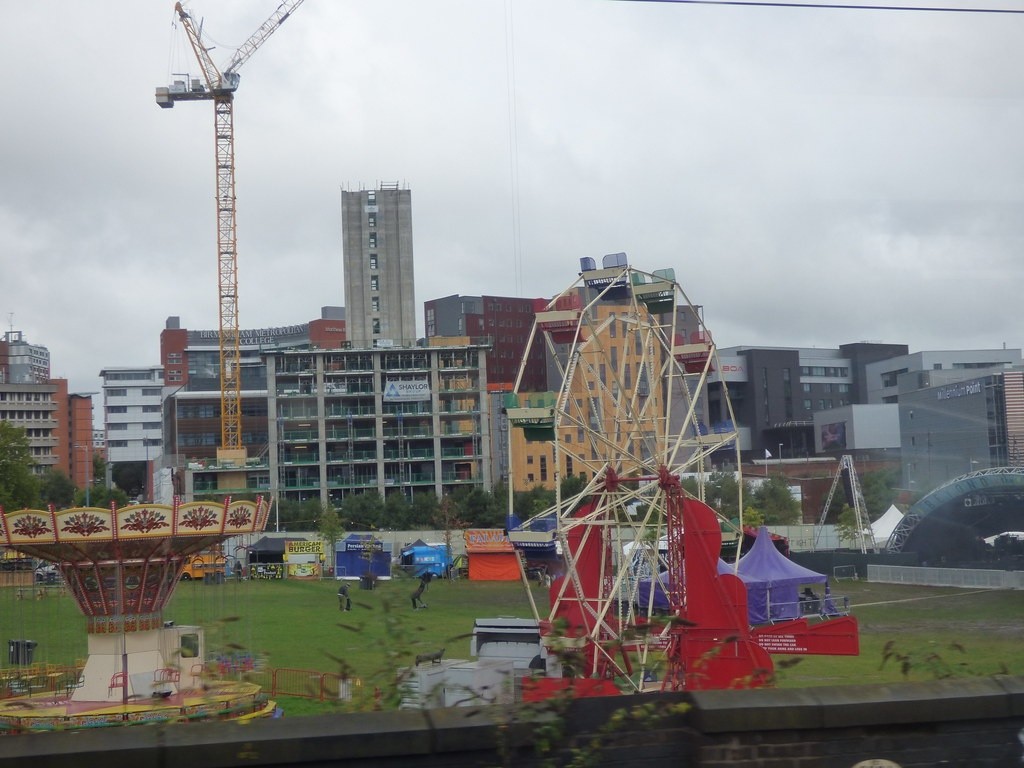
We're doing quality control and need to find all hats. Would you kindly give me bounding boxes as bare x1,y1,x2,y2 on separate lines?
346,583,350,586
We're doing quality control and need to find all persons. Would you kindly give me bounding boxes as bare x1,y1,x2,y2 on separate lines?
233,561,243,583
336,658,353,703
412,572,431,609
801,587,820,613
537,572,557,591
337,583,351,612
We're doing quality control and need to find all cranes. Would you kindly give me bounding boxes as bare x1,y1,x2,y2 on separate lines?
153,1,311,467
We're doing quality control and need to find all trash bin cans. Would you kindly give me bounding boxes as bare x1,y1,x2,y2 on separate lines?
203,569,225,586
6,636,39,667
357,574,377,590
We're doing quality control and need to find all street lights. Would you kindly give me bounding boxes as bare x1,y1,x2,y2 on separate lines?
907,462,915,491
778,444,784,462
970,456,978,472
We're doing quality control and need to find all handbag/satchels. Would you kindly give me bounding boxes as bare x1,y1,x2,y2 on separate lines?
346,599,352,610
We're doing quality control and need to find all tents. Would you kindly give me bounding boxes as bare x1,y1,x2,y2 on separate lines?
639,555,767,624
727,527,828,623
401,539,429,564
865,504,905,546
